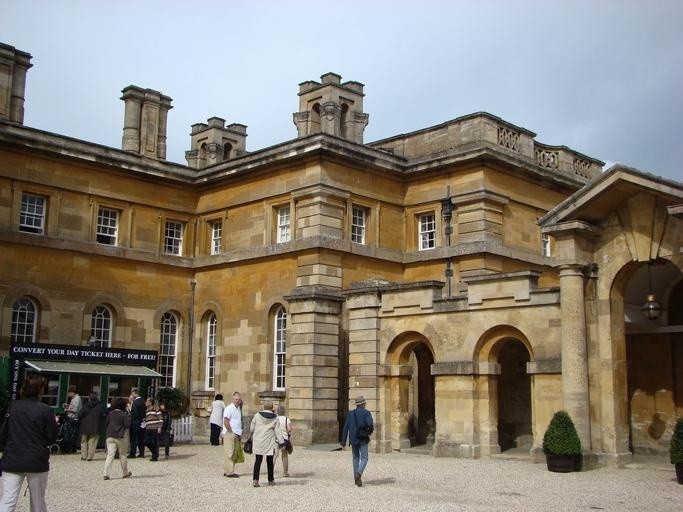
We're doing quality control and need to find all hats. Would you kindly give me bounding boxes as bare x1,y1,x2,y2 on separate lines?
353,395,367,406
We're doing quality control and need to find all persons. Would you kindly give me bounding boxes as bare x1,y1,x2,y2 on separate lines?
62,387,82,424
271,404,291,478
338,395,374,488
139,397,164,462
206,393,225,446
223,391,245,478
249,401,287,488
77,391,103,462
0,373,59,512
158,401,173,459
126,386,145,459
103,398,132,481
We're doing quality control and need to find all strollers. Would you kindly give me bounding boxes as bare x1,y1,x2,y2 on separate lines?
49,411,75,456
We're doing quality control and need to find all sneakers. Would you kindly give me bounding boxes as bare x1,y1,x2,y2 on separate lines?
121,470,132,478
165,455,169,460
282,472,289,477
102,475,110,480
352,472,362,487
148,457,157,461
222,472,239,478
126,454,135,458
136,454,144,458
80,457,91,461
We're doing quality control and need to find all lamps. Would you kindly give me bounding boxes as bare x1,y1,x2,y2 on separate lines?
640,262,664,321
86,336,97,344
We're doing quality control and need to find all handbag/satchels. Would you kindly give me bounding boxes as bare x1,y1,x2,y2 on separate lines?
284,440,293,455
243,439,254,454
355,424,374,444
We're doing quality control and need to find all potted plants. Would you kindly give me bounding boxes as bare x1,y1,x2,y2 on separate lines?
155,386,186,447
542,410,582,473
669,417,683,485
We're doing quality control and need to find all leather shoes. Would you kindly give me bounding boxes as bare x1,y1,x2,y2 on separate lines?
252,482,260,488
267,480,275,486
